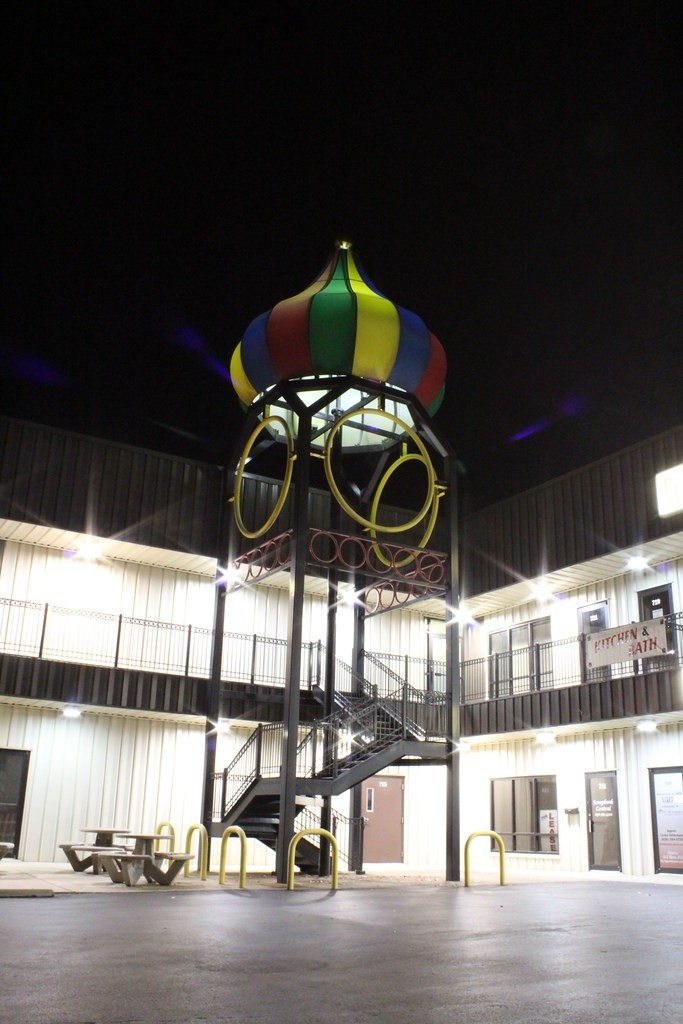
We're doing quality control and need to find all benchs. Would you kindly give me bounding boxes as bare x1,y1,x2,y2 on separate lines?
58,842,196,888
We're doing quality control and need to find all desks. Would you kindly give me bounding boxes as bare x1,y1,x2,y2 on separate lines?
115,834,173,881
78,828,130,874
